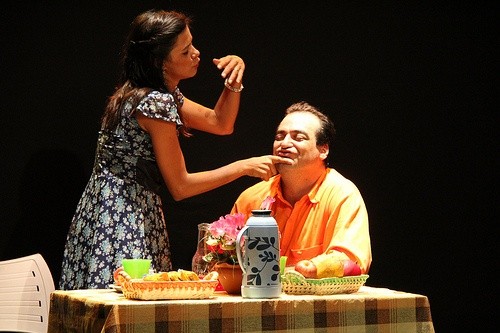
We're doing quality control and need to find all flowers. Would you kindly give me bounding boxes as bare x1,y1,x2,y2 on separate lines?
198,196,281,278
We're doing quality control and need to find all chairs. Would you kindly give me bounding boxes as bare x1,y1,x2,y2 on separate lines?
0,253,55,333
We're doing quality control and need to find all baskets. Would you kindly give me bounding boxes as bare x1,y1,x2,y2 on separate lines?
280,270,369,295
117,271,219,301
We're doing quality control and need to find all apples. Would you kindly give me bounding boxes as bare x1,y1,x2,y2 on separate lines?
343,259,361,277
295,260,317,278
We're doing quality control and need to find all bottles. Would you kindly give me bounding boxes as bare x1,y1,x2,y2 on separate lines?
192,222,211,279
235,209,282,299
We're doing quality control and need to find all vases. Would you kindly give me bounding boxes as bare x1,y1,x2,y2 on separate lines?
215,263,242,295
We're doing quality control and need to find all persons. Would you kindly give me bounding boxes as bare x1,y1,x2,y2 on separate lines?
58,9,293,292
229,100,373,276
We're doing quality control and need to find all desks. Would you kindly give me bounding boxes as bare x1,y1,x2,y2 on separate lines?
47,286,435,333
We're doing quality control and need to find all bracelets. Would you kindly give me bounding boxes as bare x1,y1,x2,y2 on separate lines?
224,77,244,92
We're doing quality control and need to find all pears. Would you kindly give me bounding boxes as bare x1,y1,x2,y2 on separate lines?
316,257,344,279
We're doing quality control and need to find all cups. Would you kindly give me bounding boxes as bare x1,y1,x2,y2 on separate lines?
122,258,153,279
280,255,288,282
